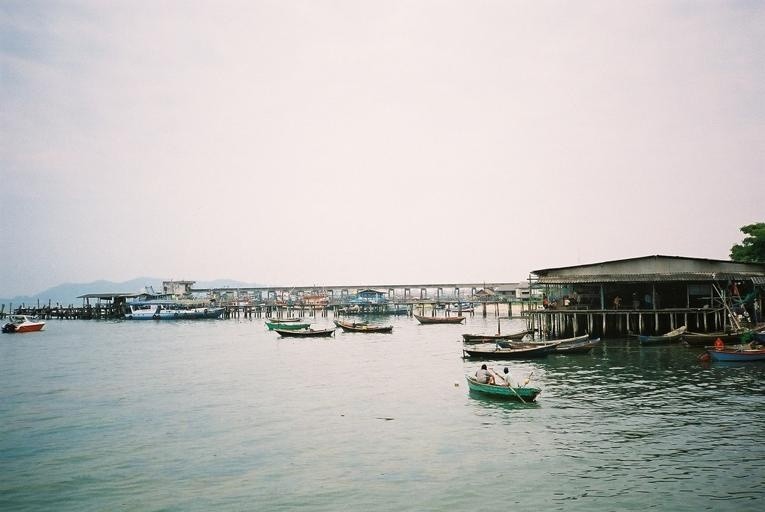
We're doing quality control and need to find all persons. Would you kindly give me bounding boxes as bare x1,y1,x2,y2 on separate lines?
713,337,725,352
495,367,512,387
542,292,582,310
612,295,623,310
474,364,496,386
521,332,534,342
644,292,652,310
654,291,662,309
728,281,740,297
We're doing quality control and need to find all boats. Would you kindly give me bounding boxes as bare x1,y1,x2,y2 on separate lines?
1,316,47,332
124,300,226,320
413,313,466,324
264,316,335,337
331,318,394,333
639,325,764,362
464,373,542,403
461,328,600,358
